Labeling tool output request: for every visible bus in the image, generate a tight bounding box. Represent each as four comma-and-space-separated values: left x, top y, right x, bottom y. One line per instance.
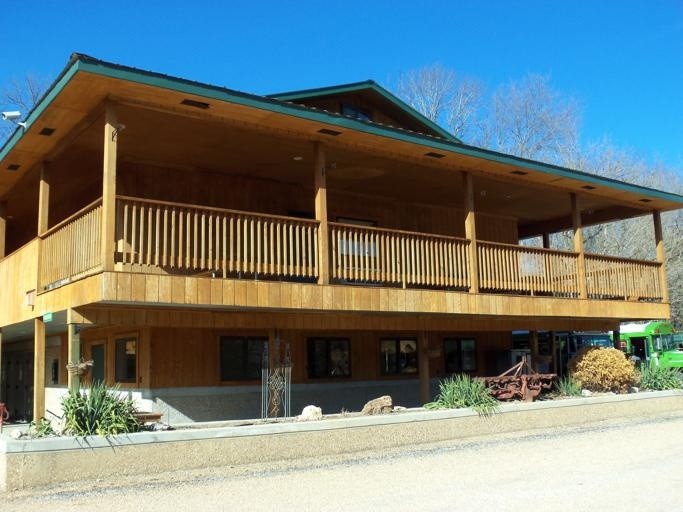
512, 320, 683, 388
